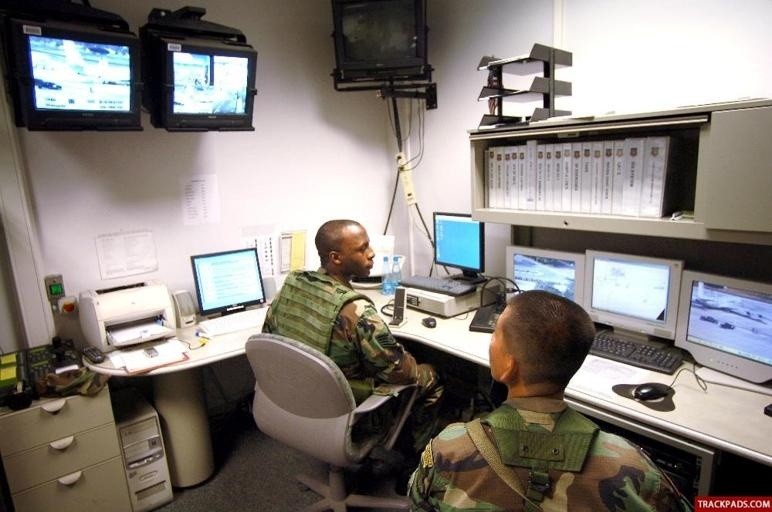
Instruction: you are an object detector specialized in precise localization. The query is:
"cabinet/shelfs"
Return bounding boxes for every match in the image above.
[470,97,772,238]
[1,385,134,512]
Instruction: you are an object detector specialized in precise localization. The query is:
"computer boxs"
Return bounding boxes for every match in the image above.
[116,390,175,512]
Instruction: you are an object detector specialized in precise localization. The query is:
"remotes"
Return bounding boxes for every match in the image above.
[82,345,104,363]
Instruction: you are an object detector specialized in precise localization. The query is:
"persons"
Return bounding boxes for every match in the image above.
[261,221,448,497]
[407,289,695,512]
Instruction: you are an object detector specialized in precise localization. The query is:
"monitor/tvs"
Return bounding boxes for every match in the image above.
[1,23,144,131]
[191,248,267,315]
[145,35,258,132]
[431,211,484,274]
[584,248,683,340]
[505,246,584,310]
[675,268,772,397]
[332,1,426,80]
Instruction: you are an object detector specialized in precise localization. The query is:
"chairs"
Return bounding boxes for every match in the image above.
[244,333,422,512]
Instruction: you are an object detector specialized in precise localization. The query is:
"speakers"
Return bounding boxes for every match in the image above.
[173,287,201,325]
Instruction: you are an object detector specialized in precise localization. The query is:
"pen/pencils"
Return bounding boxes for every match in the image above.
[194,332,212,340]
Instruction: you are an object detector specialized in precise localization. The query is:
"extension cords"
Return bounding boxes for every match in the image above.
[397,153,418,207]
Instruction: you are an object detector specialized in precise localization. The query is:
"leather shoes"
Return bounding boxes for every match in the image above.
[395,466,415,496]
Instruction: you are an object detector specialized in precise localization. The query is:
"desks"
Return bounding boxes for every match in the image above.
[82,287,770,469]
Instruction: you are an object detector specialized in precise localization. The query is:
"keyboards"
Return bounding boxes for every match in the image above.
[198,308,268,336]
[588,333,685,375]
[399,274,477,296]
[469,301,503,333]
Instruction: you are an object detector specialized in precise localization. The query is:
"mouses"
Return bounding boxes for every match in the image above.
[424,315,438,329]
[637,383,673,401]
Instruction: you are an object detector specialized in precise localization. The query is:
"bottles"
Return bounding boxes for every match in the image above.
[381,256,402,296]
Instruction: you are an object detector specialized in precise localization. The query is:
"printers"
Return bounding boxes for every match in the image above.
[80,279,188,374]
